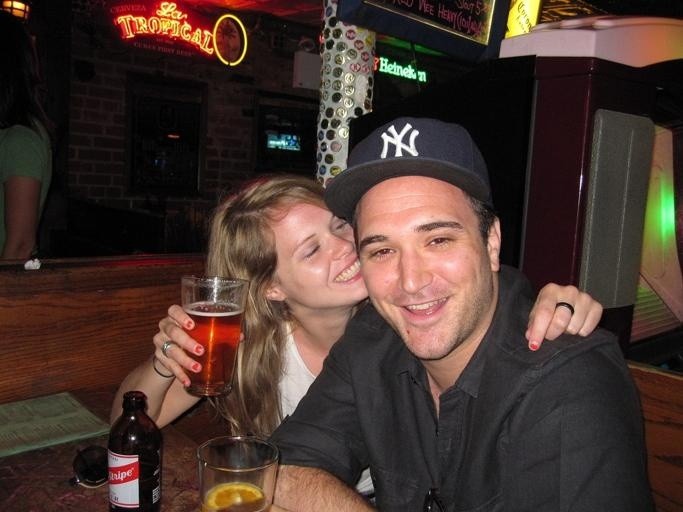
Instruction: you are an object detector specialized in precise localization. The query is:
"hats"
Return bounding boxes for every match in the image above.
[324,116,494,222]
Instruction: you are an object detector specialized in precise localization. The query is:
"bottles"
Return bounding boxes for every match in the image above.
[106,390,164,509]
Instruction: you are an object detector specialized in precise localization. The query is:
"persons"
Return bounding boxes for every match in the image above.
[0,35,53,259]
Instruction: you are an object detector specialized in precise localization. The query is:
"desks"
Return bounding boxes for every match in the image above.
[0,381,290,510]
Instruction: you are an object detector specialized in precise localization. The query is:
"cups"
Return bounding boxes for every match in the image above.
[179,276,251,399]
[196,433,280,512]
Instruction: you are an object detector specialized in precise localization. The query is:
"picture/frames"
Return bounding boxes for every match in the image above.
[122,73,208,200]
